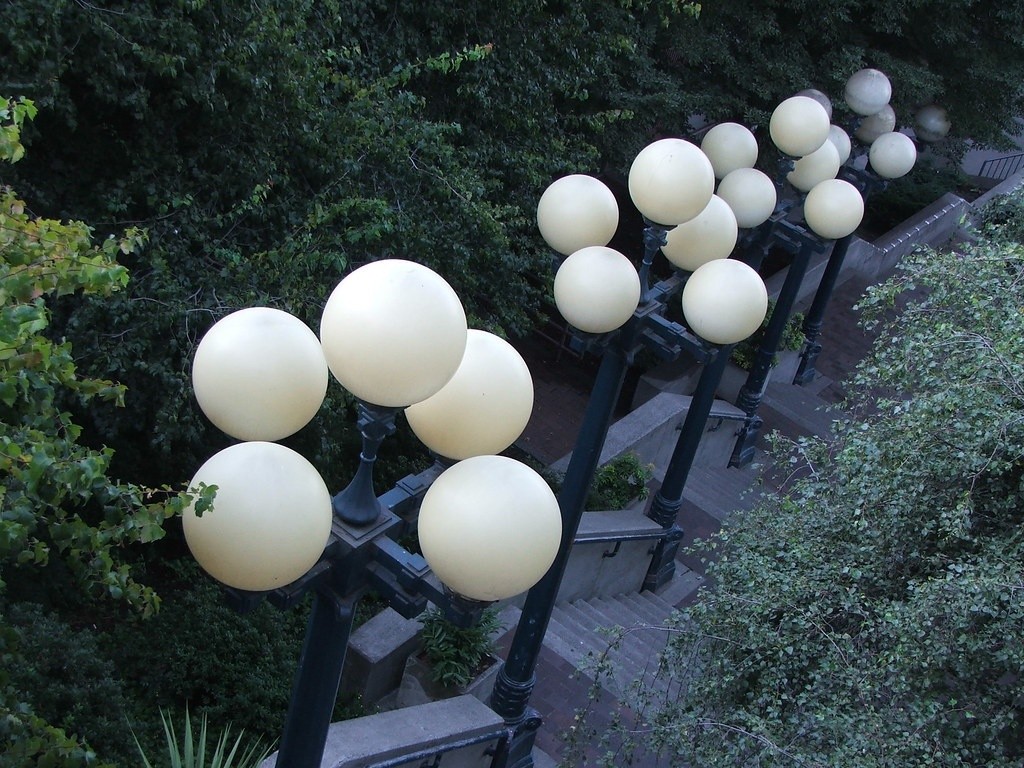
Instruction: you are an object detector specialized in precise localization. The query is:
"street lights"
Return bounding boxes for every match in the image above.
[482,68,951,768]
[183,259,563,768]
